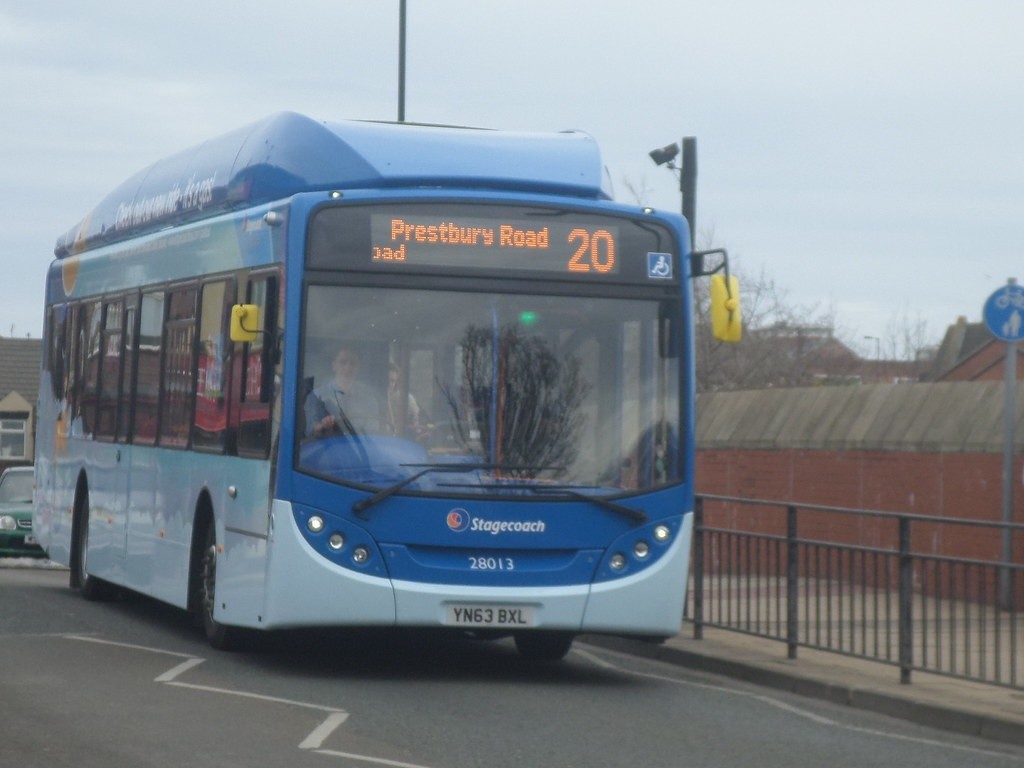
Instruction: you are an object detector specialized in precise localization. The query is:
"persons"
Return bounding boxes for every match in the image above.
[385,363,422,438]
[303,347,382,443]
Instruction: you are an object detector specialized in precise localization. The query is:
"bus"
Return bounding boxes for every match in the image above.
[31,113,740,661]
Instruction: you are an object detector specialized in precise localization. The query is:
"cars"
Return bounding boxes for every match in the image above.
[0,468,53,559]
